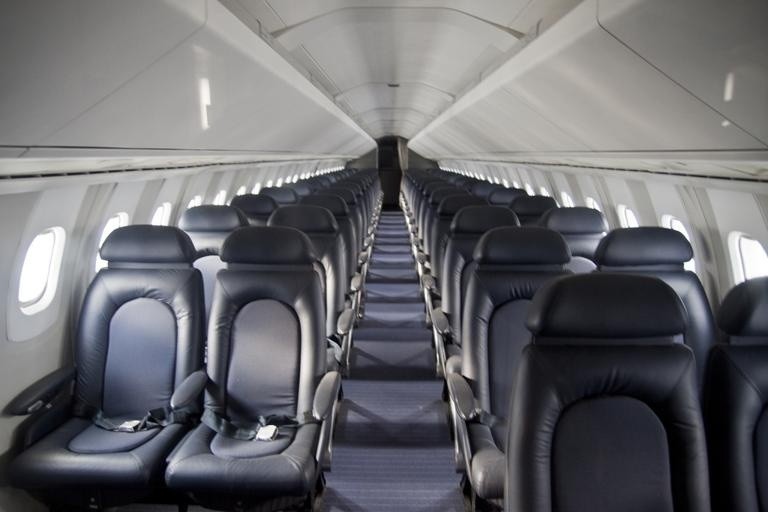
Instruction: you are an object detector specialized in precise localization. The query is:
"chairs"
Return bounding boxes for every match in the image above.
[398,165,767,512]
[0,165,385,512]
